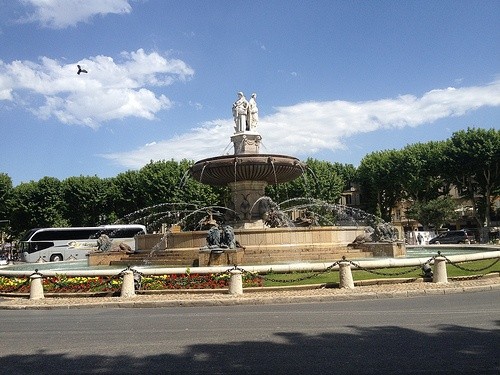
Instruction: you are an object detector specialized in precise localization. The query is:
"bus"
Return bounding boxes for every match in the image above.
[18,224,147,263]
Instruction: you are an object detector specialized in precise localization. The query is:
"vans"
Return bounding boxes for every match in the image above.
[429,231,476,244]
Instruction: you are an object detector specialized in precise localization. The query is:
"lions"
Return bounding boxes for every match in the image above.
[88,234,132,253]
[352,222,403,243]
[199,224,246,250]
